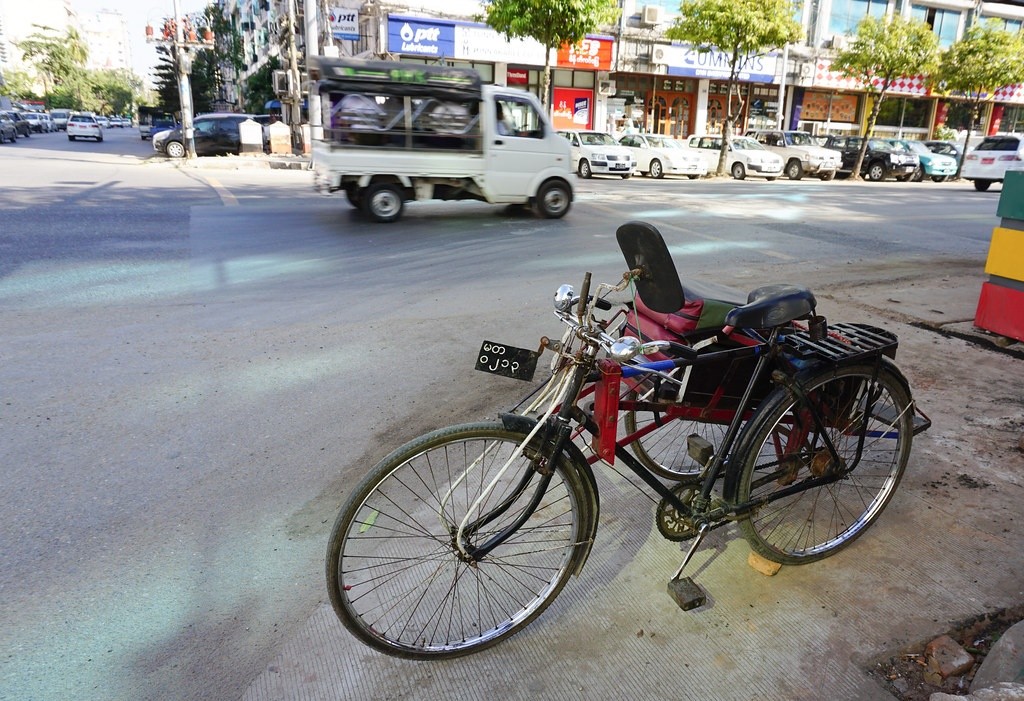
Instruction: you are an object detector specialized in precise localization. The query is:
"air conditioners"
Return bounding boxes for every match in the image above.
[639,3,665,26]
[796,62,816,79]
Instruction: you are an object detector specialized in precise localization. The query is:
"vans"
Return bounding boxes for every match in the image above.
[138,105,162,139]
[960,134,1024,191]
[685,133,784,181]
[152,112,256,152]
[813,134,920,182]
[156,117,265,156]
[52,111,71,130]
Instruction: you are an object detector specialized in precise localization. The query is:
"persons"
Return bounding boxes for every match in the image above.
[327,90,478,149]
[496,101,514,137]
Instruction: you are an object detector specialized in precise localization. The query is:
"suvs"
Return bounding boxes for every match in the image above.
[742,128,843,181]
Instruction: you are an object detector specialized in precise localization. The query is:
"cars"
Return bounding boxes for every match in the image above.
[924,141,975,180]
[0,111,18,144]
[42,114,58,131]
[122,118,133,127]
[67,114,104,141]
[96,116,110,129]
[618,133,709,179]
[22,112,49,132]
[8,111,32,137]
[554,129,638,179]
[110,117,124,127]
[886,138,958,182]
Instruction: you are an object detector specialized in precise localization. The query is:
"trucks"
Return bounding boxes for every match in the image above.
[310,52,577,221]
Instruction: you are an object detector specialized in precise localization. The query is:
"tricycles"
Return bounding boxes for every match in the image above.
[325,220,933,660]
